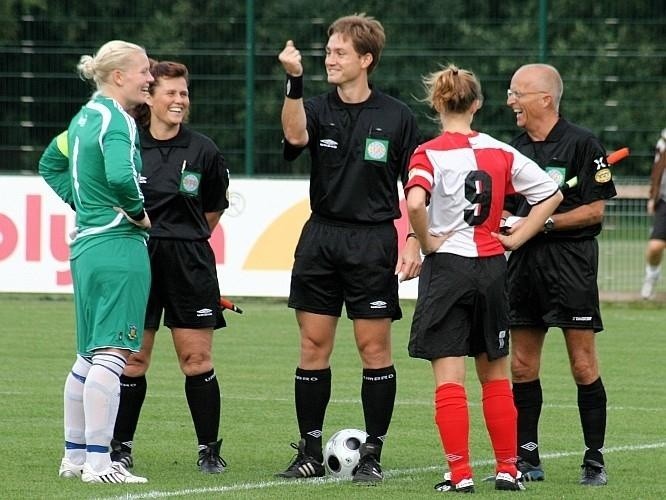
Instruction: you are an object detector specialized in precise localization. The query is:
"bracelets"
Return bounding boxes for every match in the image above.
[285,73,303,99]
[406,233,417,241]
[500,218,506,222]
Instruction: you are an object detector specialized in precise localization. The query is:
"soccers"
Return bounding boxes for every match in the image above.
[324,429,368,477]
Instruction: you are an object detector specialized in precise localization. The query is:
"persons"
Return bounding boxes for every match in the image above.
[404,64,565,493]
[481,63,617,485]
[275,13,429,483]
[110,61,230,475]
[640,128,666,300]
[38,40,155,484]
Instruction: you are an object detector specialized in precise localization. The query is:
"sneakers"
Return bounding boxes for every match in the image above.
[354,443,384,482]
[581,460,608,485]
[434,456,544,493]
[274,439,325,478]
[59,440,148,484]
[197,438,226,473]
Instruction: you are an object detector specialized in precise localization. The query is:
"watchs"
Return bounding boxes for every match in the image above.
[544,217,554,234]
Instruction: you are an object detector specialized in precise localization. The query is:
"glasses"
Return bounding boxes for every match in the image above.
[507,89,548,97]
